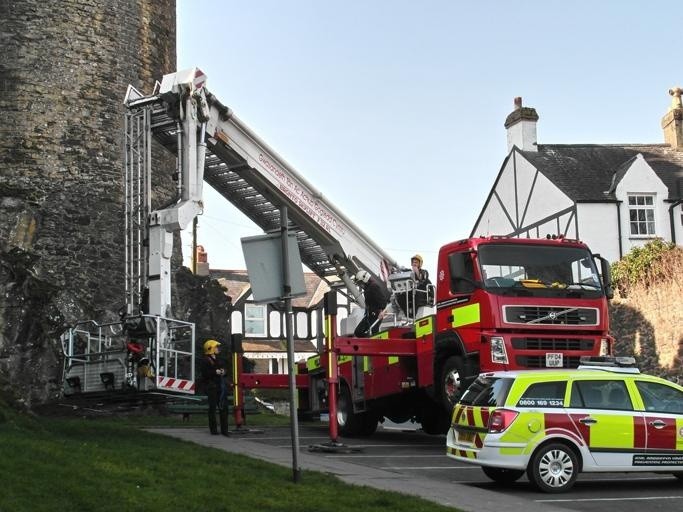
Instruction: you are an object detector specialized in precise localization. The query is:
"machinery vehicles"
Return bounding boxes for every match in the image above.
[57,67,616,438]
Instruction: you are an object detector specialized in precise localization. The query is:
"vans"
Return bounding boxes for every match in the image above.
[445,367,683,494]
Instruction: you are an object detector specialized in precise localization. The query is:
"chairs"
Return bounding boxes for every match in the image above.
[584,388,629,408]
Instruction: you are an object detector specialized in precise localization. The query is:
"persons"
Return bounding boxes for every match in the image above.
[397,255,433,318]
[202,339,231,437]
[354,271,387,338]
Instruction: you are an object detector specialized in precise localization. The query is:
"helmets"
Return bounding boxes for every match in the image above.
[203,340,222,355]
[411,255,423,266]
[355,271,371,283]
